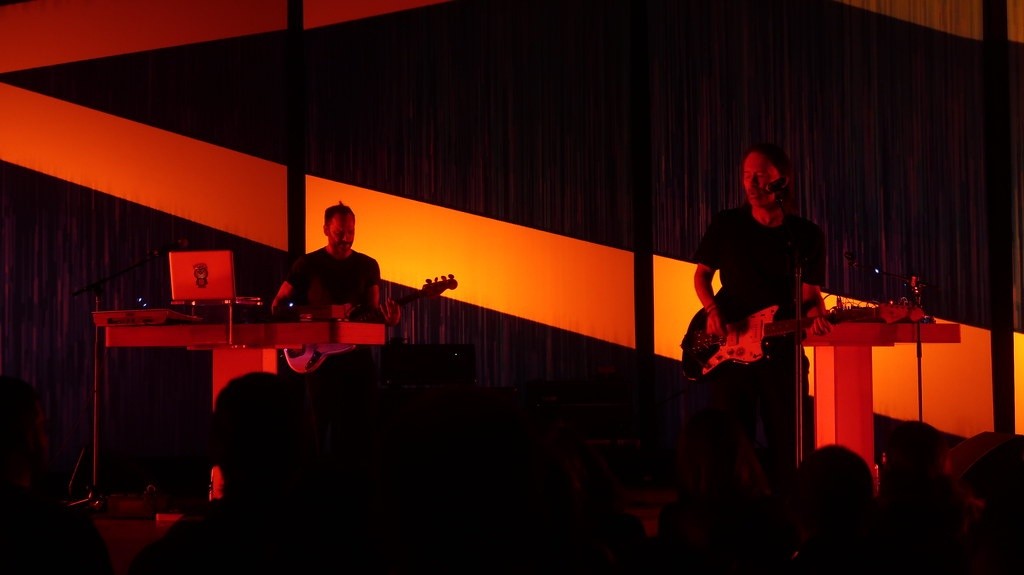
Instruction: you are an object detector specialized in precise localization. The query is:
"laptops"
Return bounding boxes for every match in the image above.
[169,251,261,301]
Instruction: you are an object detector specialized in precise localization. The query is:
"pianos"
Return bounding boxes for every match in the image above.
[104,322,386,348]
[798,323,962,347]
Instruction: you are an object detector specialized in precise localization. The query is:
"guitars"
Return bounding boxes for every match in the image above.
[681,296,913,382]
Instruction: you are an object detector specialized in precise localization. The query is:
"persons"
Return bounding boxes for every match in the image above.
[127,375,1024,575]
[694,144,831,491]
[272,204,402,450]
[0,375,113,575]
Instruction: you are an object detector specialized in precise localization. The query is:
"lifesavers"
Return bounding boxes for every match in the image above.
[282,273,457,373]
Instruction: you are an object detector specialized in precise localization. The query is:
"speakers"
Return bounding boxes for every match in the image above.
[368,385,525,501]
[942,430,1024,517]
[536,405,640,506]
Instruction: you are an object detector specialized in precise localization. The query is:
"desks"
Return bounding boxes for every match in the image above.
[105,322,387,503]
[802,322,961,497]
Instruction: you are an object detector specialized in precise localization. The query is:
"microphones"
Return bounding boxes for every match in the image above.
[766,176,789,192]
[154,239,188,256]
